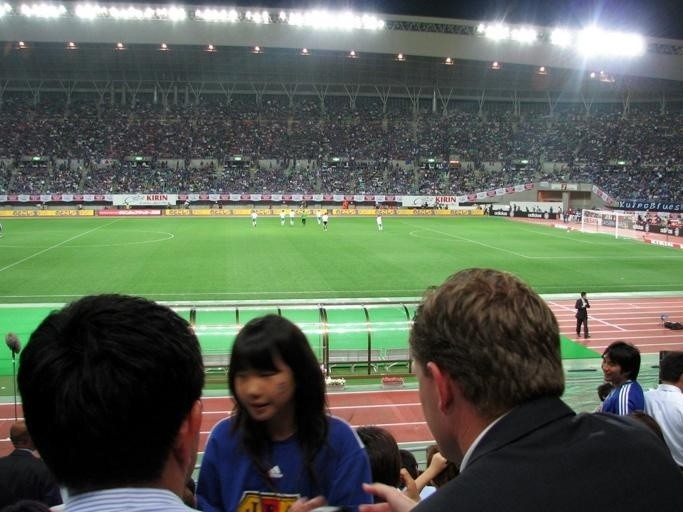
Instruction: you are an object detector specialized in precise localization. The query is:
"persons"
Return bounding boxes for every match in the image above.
[573,291,593,339]
[0,96,682,227]
[642,352,682,469]
[594,340,644,418]
[320,212,329,232]
[0,420,68,512]
[594,382,614,412]
[196,313,374,512]
[352,263,683,512]
[375,214,383,231]
[356,425,460,503]
[15,290,208,512]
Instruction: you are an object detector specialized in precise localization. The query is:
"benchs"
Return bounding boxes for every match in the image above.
[200,348,414,375]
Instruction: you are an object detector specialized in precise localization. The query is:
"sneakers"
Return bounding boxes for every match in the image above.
[584,335,591,339]
[577,332,581,337]
